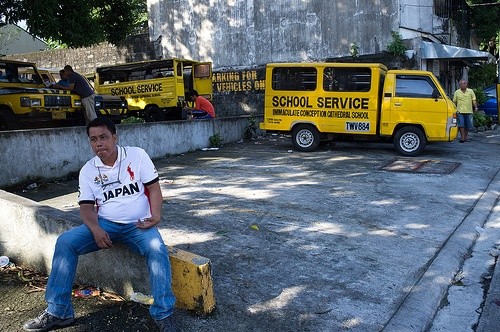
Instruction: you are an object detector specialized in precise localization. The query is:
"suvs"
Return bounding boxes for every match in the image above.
[1,59,82,128]
[38,69,128,124]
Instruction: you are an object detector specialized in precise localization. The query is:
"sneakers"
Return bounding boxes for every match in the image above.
[154,314,184,332]
[23,311,75,332]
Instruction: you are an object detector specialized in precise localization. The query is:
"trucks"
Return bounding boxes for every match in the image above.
[86,57,214,124]
[259,63,458,156]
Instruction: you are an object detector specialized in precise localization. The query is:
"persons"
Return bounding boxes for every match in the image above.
[22,116,179,332]
[453,79,478,143]
[182,90,215,120]
[50,66,97,121]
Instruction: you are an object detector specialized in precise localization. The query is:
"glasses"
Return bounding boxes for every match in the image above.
[101,180,122,190]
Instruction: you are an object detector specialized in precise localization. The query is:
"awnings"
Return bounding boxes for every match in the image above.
[419,41,497,62]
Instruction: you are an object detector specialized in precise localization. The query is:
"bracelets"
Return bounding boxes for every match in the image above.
[475,108,478,109]
